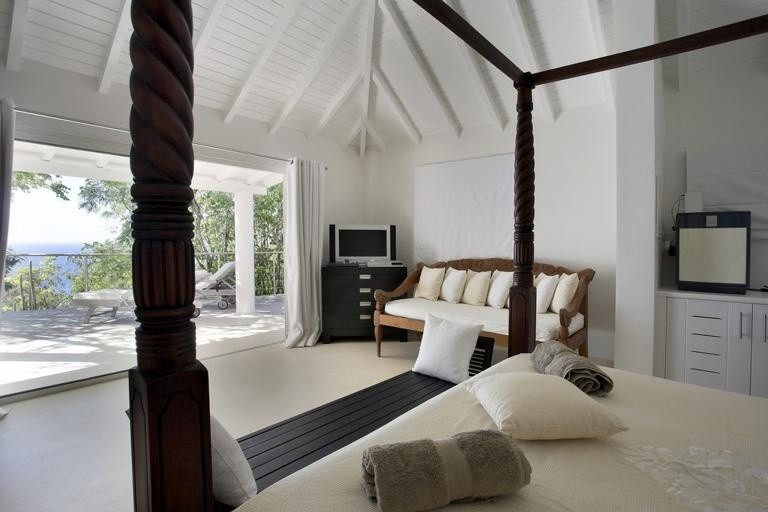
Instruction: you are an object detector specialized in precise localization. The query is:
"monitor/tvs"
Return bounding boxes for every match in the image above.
[328,224,396,268]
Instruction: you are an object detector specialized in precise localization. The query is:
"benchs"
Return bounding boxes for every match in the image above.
[214,335,495,512]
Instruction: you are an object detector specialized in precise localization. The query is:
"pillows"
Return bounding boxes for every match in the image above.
[486,269,514,308]
[439,267,466,304]
[411,312,484,385]
[463,373,630,439]
[548,272,579,314]
[414,265,445,301]
[505,274,536,308]
[209,414,258,507]
[460,269,491,306]
[534,272,561,313]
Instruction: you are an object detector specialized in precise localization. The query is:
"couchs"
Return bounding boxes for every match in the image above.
[371,258,596,358]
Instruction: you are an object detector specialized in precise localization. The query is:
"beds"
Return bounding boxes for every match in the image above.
[129,0,768,512]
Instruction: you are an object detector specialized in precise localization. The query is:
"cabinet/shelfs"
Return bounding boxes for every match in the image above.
[321,263,407,342]
[666,296,768,399]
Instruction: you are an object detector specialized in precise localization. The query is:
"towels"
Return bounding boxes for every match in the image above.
[359,427,532,512]
[530,340,613,398]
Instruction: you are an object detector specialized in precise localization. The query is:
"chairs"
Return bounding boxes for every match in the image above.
[73,260,235,323]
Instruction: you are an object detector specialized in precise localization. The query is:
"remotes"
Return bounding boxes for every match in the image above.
[326,261,359,268]
